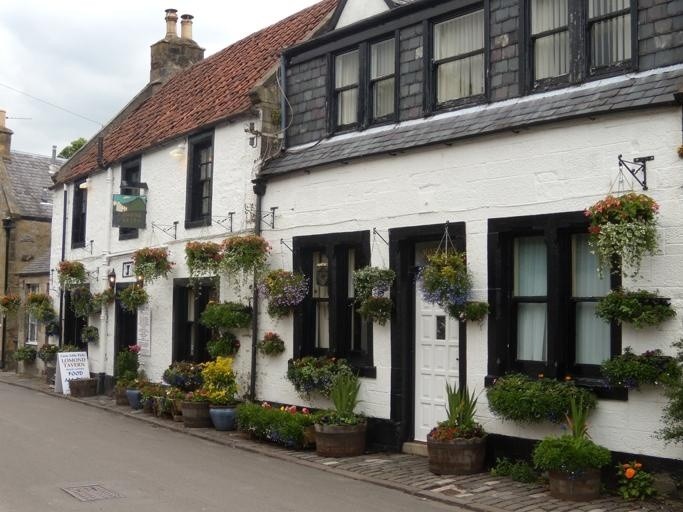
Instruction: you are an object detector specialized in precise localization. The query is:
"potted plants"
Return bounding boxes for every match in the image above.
[534,438,611,501]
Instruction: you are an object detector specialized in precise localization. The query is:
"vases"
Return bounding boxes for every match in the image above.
[126,387,238,432]
[66,376,97,397]
[315,422,366,456]
[424,433,487,473]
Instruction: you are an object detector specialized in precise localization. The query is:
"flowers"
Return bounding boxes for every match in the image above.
[314,371,367,426]
[414,249,470,308]
[115,235,310,404]
[583,193,662,279]
[424,383,487,440]
[351,265,395,306]
